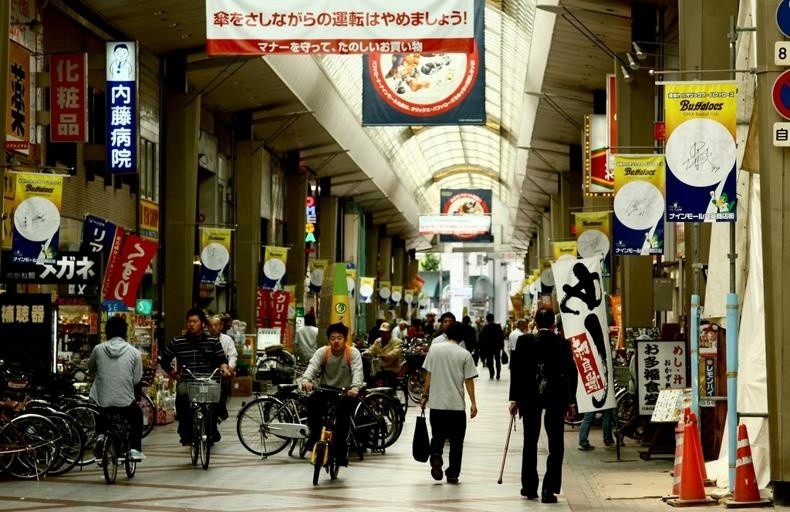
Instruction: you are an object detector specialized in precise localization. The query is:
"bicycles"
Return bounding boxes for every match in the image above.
[562,325,656,442]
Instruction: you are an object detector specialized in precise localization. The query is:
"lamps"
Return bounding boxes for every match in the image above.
[618,36,680,84]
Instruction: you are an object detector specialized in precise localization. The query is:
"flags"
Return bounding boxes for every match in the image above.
[548,256,619,415]
[105,232,161,308]
[611,151,666,257]
[308,258,428,310]
[197,226,233,284]
[656,79,740,223]
[518,237,576,300]
[572,210,614,279]
[261,245,288,291]
[11,171,63,261]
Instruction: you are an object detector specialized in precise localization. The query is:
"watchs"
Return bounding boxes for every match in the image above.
[420,393,431,399]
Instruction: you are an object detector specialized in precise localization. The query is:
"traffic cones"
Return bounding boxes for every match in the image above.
[663,409,717,508]
[725,423,775,509]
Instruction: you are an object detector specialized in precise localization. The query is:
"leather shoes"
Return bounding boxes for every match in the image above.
[541,488,560,503]
[520,486,539,499]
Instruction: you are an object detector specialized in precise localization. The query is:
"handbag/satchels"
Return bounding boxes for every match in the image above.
[411,408,432,464]
[501,351,509,365]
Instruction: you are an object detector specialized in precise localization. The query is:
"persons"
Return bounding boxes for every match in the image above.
[430,312,467,443]
[358,321,405,415]
[208,311,238,423]
[366,312,529,380]
[625,351,648,436]
[296,320,365,466]
[505,307,577,502]
[579,409,623,454]
[84,315,146,462]
[293,313,319,364]
[419,319,477,486]
[157,308,231,446]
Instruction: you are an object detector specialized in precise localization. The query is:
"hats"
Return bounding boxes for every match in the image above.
[379,322,393,333]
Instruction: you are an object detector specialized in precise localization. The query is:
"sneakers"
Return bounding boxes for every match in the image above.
[92,434,106,458]
[605,441,620,450]
[335,453,348,467]
[430,453,443,481]
[578,439,595,451]
[445,469,460,484]
[306,429,321,452]
[177,425,224,446]
[124,448,147,460]
[475,363,502,380]
